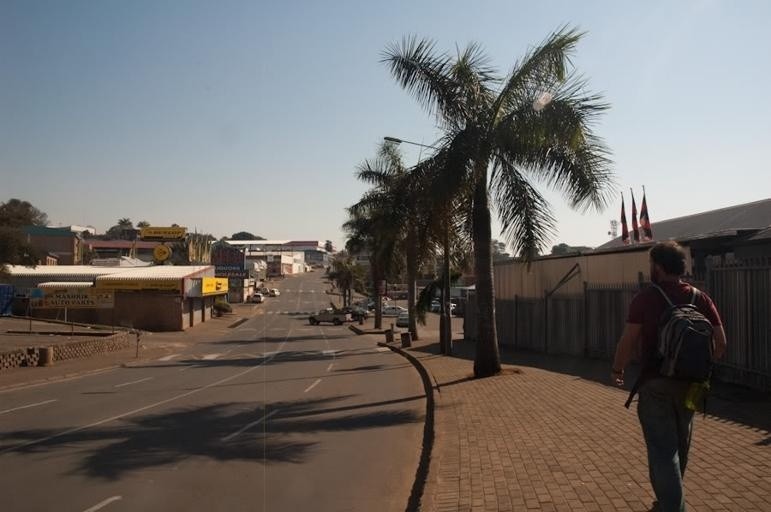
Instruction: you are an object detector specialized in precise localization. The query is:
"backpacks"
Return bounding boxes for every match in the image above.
[650,283,715,393]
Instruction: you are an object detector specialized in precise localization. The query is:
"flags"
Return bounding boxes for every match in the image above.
[620,193,632,247]
[640,190,654,242]
[631,192,642,245]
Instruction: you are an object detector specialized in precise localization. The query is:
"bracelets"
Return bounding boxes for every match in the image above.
[612,367,626,376]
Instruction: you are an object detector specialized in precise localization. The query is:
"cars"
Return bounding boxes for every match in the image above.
[252,287,280,304]
[339,294,409,327]
[427,299,456,313]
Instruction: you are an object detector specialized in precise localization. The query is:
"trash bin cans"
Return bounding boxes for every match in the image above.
[39,347,53,366]
[400,332,412,347]
[385,330,393,343]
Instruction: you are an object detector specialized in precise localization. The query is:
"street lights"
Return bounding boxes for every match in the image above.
[382,135,455,357]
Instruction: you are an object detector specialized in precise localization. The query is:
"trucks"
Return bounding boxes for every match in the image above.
[309,308,352,325]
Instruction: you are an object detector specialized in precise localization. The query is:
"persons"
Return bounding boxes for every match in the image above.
[610,241,730,511]
